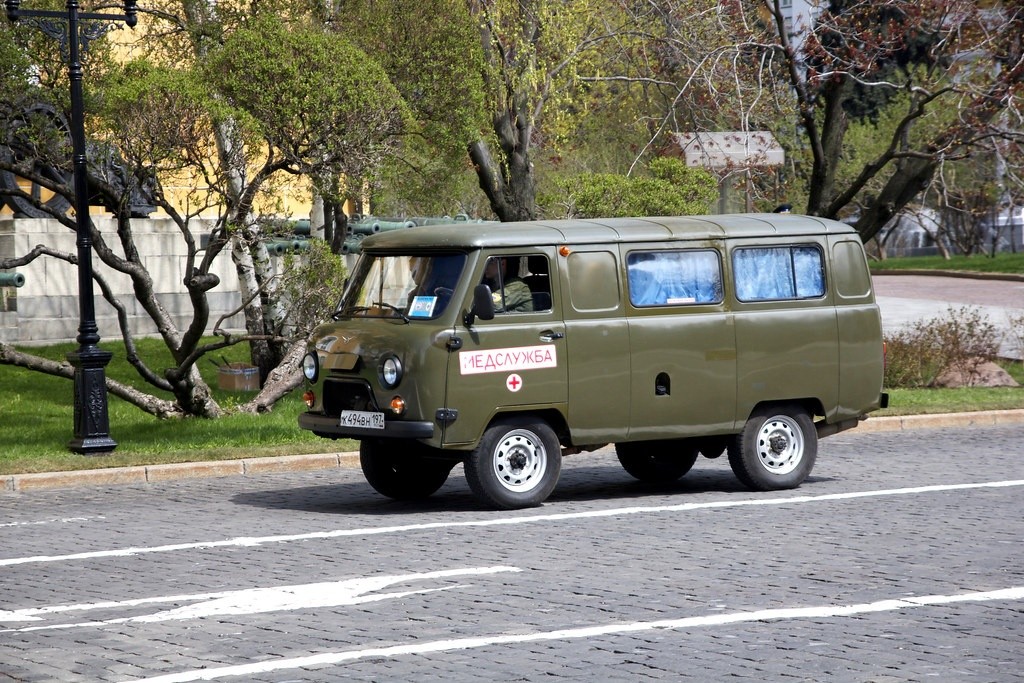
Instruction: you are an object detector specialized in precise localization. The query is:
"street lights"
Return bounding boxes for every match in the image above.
[6,0,137,454]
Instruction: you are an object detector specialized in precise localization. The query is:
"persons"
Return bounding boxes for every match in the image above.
[401,256,450,317]
[472,257,533,312]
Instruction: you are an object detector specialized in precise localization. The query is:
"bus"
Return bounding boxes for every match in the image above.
[297,202,889,514]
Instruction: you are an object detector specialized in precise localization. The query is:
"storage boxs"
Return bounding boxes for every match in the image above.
[216,362,260,392]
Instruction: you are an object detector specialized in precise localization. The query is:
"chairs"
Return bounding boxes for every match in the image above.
[531,291,551,311]
[524,256,549,293]
